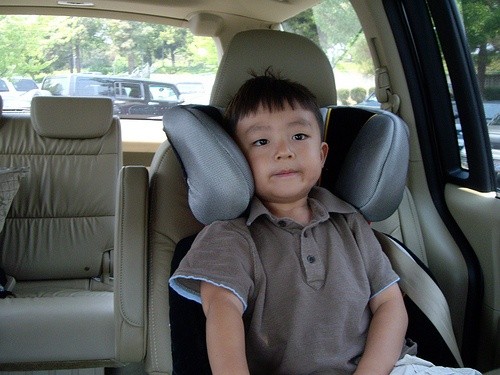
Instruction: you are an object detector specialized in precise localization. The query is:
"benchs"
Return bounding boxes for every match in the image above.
[0,96,127,364]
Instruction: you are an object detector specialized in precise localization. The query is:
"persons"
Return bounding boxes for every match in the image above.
[168,65,484,375]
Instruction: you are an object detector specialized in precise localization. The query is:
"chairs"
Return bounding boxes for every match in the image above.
[113,29,429,375]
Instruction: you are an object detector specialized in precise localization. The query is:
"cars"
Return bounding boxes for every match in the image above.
[0,76,43,110]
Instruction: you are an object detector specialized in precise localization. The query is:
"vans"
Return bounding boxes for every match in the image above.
[41,73,186,117]
